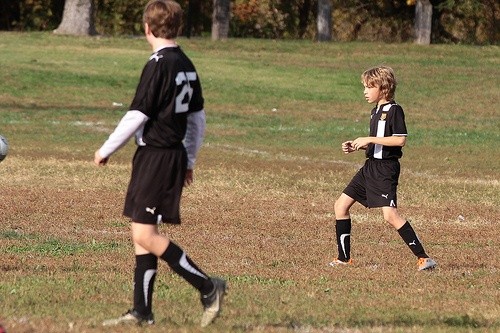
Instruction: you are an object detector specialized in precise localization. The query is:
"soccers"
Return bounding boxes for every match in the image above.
[0,135,8,164]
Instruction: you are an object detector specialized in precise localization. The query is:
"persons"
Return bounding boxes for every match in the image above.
[93,0,228,330]
[324,64,438,273]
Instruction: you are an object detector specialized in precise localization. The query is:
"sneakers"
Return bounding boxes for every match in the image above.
[103,308,155,327]
[417,257,436,271]
[200,278,227,328]
[325,258,353,267]
